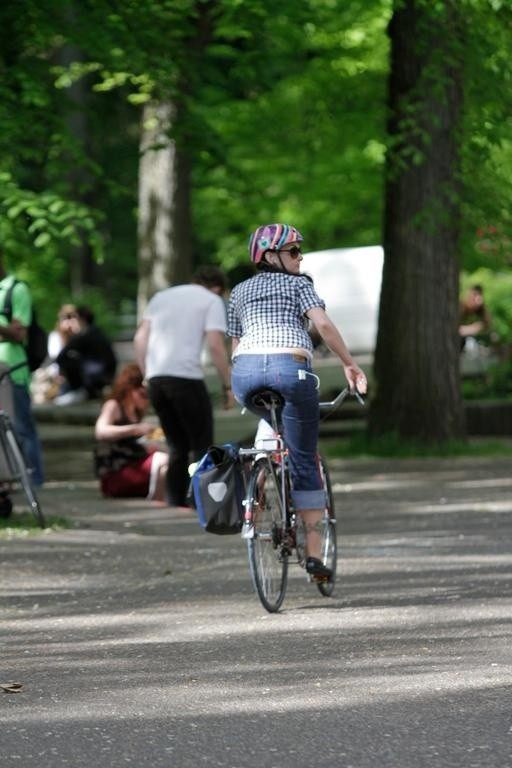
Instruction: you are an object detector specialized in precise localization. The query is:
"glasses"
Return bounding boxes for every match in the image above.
[270,247,303,260]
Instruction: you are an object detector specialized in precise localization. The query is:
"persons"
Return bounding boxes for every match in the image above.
[0,265,44,489]
[132,263,234,509]
[459,282,494,355]
[222,222,369,582]
[50,302,118,407]
[94,362,171,500]
[45,303,84,360]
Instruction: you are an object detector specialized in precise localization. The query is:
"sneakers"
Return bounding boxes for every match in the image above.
[305,556,335,580]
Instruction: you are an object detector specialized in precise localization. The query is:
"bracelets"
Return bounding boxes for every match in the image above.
[223,383,233,391]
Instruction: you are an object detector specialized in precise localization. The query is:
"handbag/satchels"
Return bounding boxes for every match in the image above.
[186,441,248,536]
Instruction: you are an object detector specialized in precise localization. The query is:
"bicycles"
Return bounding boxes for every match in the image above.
[238,385,366,613]
[0,357,46,528]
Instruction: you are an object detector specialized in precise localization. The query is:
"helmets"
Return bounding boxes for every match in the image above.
[248,223,304,265]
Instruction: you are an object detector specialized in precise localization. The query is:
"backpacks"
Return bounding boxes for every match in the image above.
[3,276,48,372]
